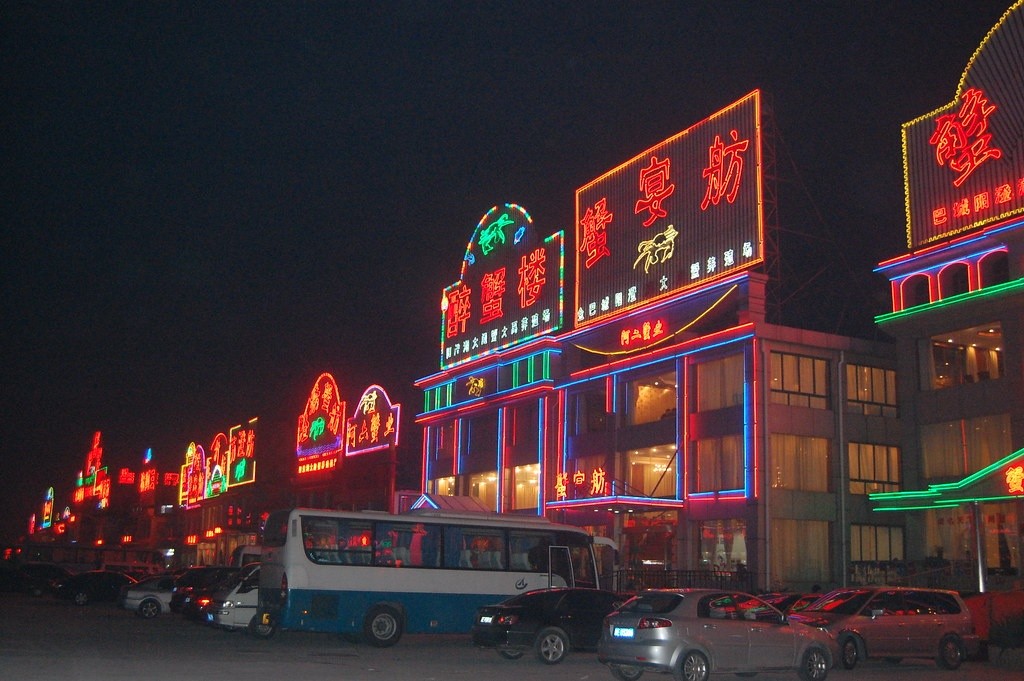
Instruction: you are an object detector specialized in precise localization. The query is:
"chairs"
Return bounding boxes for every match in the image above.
[481,551,492,568]
[511,553,530,570]
[708,607,727,618]
[461,550,473,568]
[492,551,505,569]
[343,552,352,564]
[331,545,342,563]
[353,546,372,565]
[390,547,411,566]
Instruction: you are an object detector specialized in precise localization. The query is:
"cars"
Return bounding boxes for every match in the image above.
[18,562,89,608]
[737,592,827,626]
[474,587,634,665]
[599,590,837,681]
[74,570,141,607]
[124,575,182,618]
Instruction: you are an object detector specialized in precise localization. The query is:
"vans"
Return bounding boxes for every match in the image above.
[232,544,265,569]
[101,562,162,582]
[209,560,267,632]
[789,586,981,669]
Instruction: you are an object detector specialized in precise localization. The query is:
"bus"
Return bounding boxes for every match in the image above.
[258,505,621,647]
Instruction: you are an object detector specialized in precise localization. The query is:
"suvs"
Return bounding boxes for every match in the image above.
[171,564,242,622]
